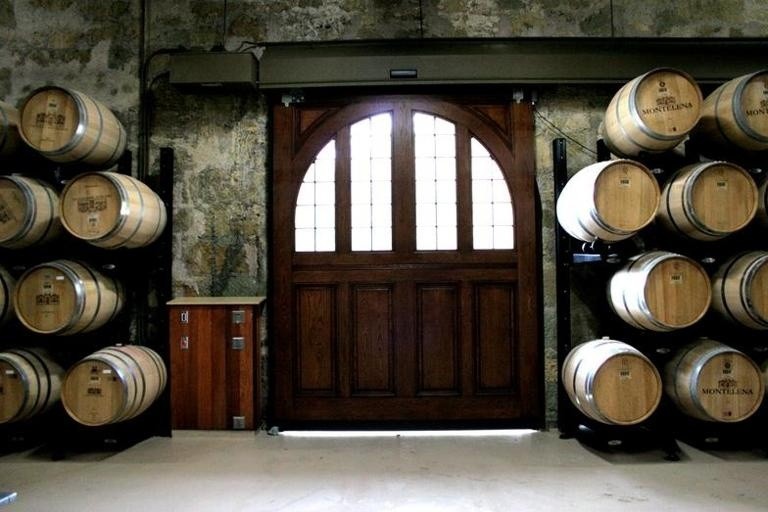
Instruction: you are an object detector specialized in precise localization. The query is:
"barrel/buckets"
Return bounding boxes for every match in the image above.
[600,67,704,158]
[12,259,127,337]
[605,249,713,332]
[0,268,18,327]
[15,83,127,170]
[555,158,662,246]
[710,249,768,332]
[59,343,170,427]
[57,171,168,251]
[756,174,768,229]
[0,175,64,251]
[695,69,768,151]
[650,160,758,243]
[0,100,22,155]
[560,336,664,427]
[0,347,67,425]
[760,357,768,396]
[658,337,765,423]
